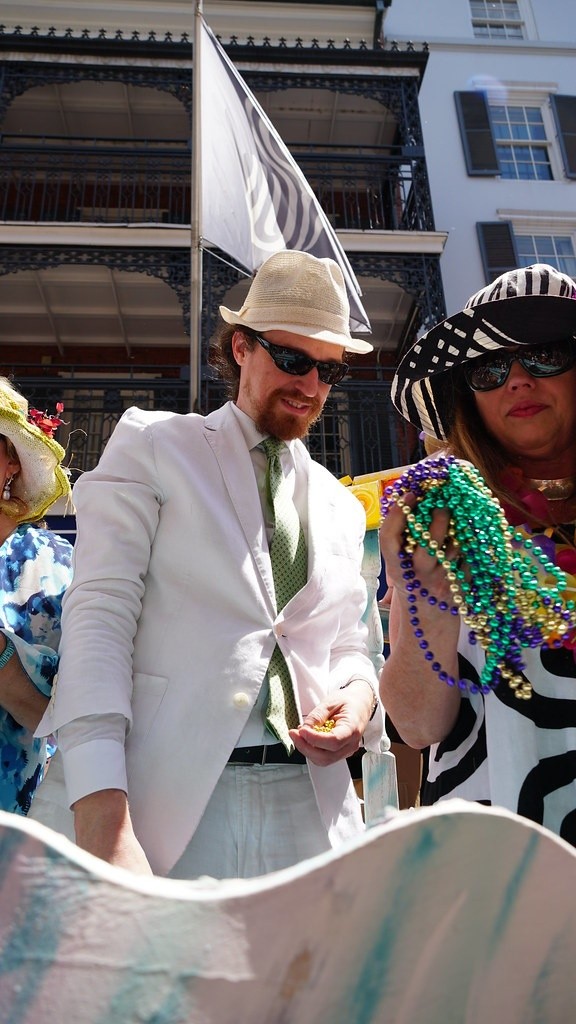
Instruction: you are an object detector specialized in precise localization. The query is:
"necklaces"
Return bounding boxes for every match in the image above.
[378,456,576,700]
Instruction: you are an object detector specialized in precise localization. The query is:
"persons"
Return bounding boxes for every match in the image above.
[0,375,75,816]
[24,247,391,882]
[378,260,576,843]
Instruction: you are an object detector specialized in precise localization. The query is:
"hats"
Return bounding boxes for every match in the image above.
[390,263,576,444]
[0,381,71,523]
[220,249,375,355]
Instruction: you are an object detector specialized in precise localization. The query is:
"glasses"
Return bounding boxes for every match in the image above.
[253,332,349,385]
[460,336,576,392]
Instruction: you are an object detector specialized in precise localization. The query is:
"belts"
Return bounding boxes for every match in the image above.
[228,743,306,764]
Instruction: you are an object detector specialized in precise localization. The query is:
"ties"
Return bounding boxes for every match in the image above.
[263,436,309,758]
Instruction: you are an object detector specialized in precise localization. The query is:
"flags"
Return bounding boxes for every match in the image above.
[199,12,375,336]
